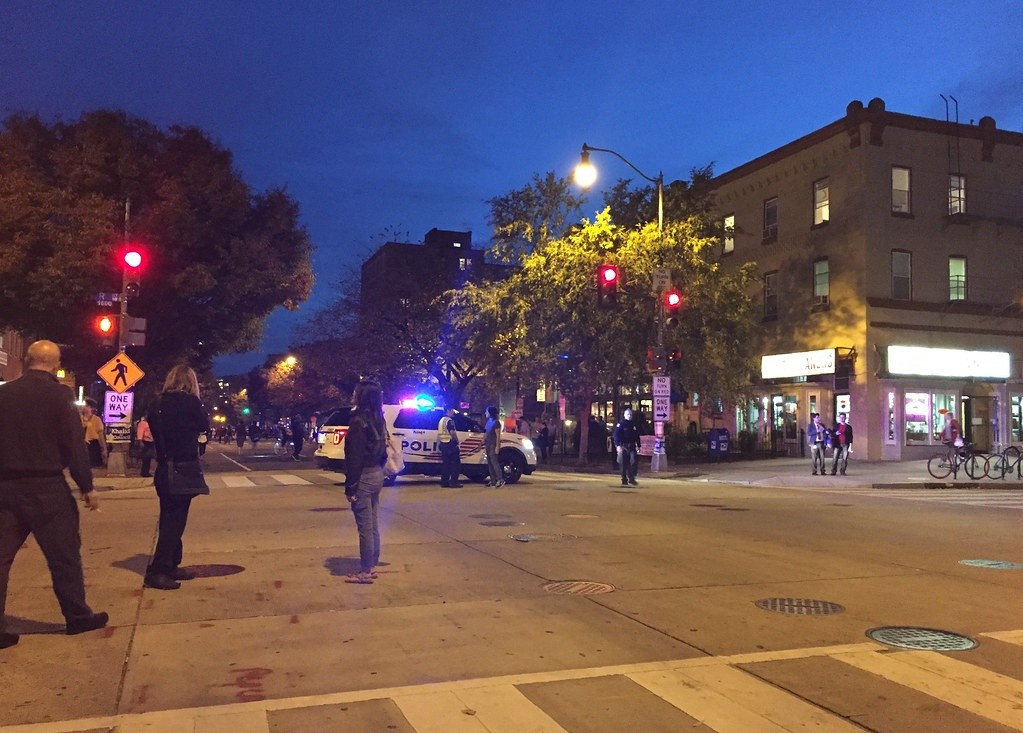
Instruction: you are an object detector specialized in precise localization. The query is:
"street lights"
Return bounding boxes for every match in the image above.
[573,144,669,473]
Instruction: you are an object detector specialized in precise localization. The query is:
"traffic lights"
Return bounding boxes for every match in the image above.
[123,249,143,296]
[121,315,147,347]
[662,291,680,330]
[96,314,119,349]
[667,347,681,368]
[647,344,664,370]
[598,265,616,309]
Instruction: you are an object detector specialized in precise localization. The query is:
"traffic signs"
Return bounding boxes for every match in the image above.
[104,391,134,423]
[653,395,670,421]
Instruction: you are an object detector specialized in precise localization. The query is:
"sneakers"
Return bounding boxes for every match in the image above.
[485,481,496,487]
[496,479,506,488]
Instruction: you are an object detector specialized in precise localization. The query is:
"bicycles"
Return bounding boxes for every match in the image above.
[273,437,295,457]
[984,444,1023,479]
[928,440,991,480]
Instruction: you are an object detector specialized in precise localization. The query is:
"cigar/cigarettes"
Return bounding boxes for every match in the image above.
[96,508,101,512]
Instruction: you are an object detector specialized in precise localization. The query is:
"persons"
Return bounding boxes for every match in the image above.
[344,380,389,585]
[207,414,317,461]
[807,413,827,475]
[80,407,155,477]
[501,416,607,464]
[938,412,961,467]
[829,412,853,475]
[614,408,641,487]
[0,340,109,649]
[437,405,462,487]
[145,364,210,591]
[483,407,505,488]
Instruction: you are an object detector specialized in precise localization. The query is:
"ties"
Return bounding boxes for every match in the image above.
[817,425,822,440]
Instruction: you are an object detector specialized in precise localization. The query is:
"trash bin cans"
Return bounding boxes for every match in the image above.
[708,427,729,458]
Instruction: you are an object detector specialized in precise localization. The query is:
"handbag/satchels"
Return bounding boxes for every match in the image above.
[383,438,405,475]
[167,439,199,474]
[954,433,965,446]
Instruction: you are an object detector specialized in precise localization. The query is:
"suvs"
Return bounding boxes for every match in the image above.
[314,398,537,484]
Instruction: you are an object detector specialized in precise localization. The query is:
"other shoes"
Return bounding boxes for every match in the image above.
[140,473,153,477]
[65,612,109,635]
[821,473,827,475]
[0,633,19,649]
[812,473,818,475]
[441,482,463,488]
[170,568,196,580]
[291,455,301,462]
[345,570,378,584]
[144,574,181,589]
[622,482,628,485]
[629,480,637,485]
[831,472,836,475]
[841,473,847,476]
